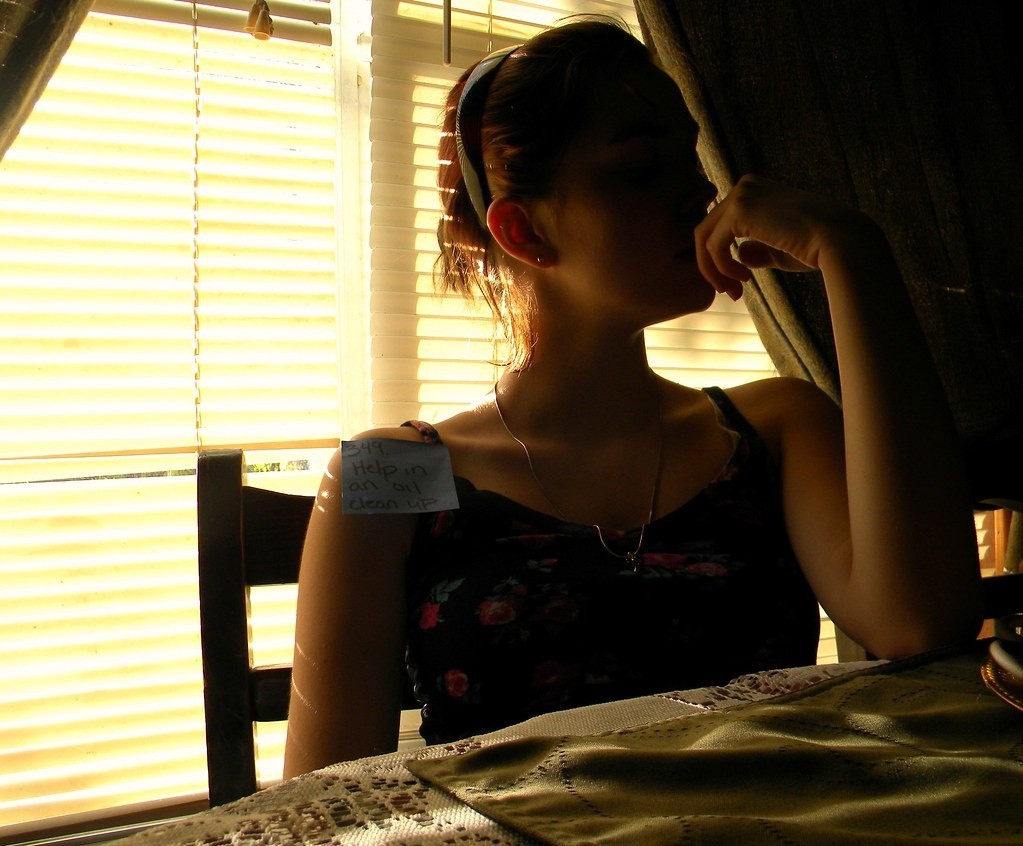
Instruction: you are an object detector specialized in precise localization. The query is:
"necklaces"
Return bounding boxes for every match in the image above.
[494,369,665,574]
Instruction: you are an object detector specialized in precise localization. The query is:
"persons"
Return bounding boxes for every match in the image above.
[285,18,987,779]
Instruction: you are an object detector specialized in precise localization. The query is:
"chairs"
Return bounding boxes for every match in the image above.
[195,455,424,809]
